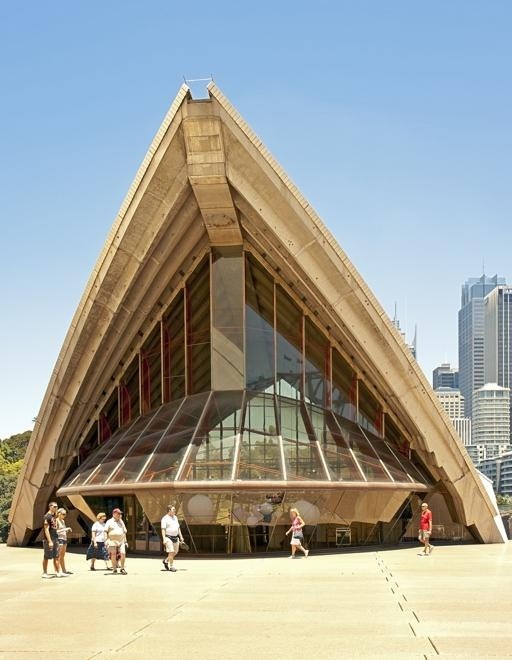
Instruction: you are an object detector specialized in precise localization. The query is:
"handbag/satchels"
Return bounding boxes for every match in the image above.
[294,531,303,539]
[167,535,179,542]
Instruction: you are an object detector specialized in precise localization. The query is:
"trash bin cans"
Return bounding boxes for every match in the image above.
[336,528,351,545]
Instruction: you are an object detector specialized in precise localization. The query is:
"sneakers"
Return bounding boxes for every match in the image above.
[305,550,309,558]
[419,545,434,556]
[288,555,295,559]
[42,570,73,578]
[163,560,177,572]
[120,569,127,575]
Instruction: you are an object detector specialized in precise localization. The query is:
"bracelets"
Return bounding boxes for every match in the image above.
[429,529,432,531]
[124,542,127,544]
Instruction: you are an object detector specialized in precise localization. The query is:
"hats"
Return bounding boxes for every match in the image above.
[113,508,123,513]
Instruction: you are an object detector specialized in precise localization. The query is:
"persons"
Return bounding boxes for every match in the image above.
[417,503,434,556]
[104,508,129,575]
[87,512,113,570]
[161,505,184,571]
[55,508,75,574]
[36,501,67,578]
[105,512,123,568]
[286,507,309,559]
